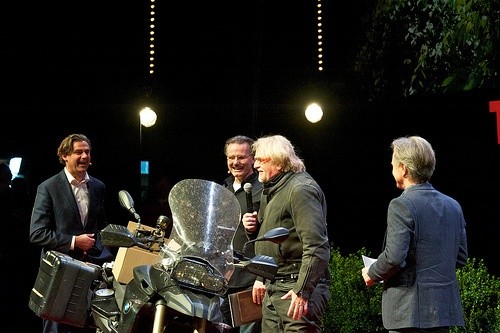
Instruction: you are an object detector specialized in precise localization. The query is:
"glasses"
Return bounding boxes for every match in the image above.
[254,158,271,164]
[226,153,252,162]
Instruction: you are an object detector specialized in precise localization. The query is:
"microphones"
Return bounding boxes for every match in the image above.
[243,182,254,214]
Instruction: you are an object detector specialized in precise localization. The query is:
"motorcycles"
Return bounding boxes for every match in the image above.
[29,178,290,333]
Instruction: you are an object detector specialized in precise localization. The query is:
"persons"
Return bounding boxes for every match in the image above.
[0,134,115,333]
[242,135,332,333]
[221,136,265,333]
[362,137,468,333]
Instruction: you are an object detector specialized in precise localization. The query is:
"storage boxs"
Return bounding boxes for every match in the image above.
[29,251,96,328]
[111,221,170,285]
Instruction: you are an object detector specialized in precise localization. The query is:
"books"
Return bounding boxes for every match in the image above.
[228,290,262,328]
